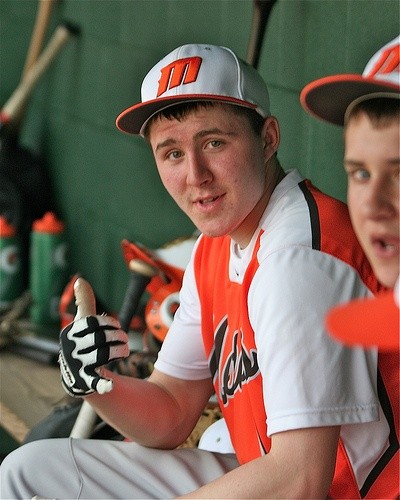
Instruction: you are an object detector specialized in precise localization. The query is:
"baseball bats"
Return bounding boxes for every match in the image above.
[67,256,159,440]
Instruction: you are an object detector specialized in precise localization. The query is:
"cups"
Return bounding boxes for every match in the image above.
[0,214,26,319]
[28,210,69,325]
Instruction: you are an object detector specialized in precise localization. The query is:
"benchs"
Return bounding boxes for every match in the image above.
[1,351,227,451]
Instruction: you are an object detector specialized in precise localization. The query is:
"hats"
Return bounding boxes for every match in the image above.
[115,43,271,138]
[299,34,399,123]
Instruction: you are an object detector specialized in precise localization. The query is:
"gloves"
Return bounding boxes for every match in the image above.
[57,277,133,398]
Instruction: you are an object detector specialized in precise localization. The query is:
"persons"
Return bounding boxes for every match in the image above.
[0,42,393,500]
[302,35,400,290]
